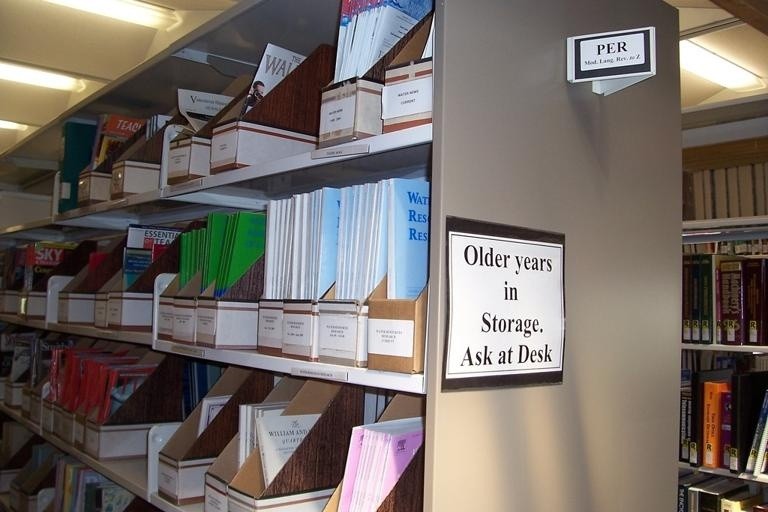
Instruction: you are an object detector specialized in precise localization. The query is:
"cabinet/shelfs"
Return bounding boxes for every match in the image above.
[680,94,767,512]
[0,0,680,512]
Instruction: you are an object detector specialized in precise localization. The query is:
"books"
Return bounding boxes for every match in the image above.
[682,239,768,345]
[58,1,435,215]
[1,178,430,300]
[693,162,768,219]
[1,322,426,512]
[680,350,768,512]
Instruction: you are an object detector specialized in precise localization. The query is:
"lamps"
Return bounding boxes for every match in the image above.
[0,120,27,133]
[677,37,764,95]
[50,0,182,30]
[0,59,86,93]
